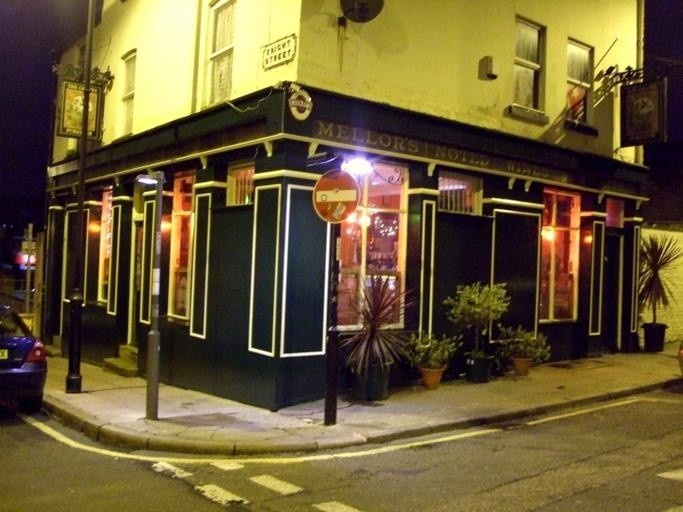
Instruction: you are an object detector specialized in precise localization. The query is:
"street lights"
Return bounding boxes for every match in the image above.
[136,171,167,419]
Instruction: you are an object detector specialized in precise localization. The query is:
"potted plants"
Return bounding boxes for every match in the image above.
[441,281,512,383]
[407,329,463,389]
[337,270,411,402]
[637,233,683,352]
[497,323,552,376]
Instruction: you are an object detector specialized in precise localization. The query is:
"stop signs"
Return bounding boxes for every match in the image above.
[312,168,360,225]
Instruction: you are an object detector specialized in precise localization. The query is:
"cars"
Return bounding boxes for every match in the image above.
[0,306,47,416]
